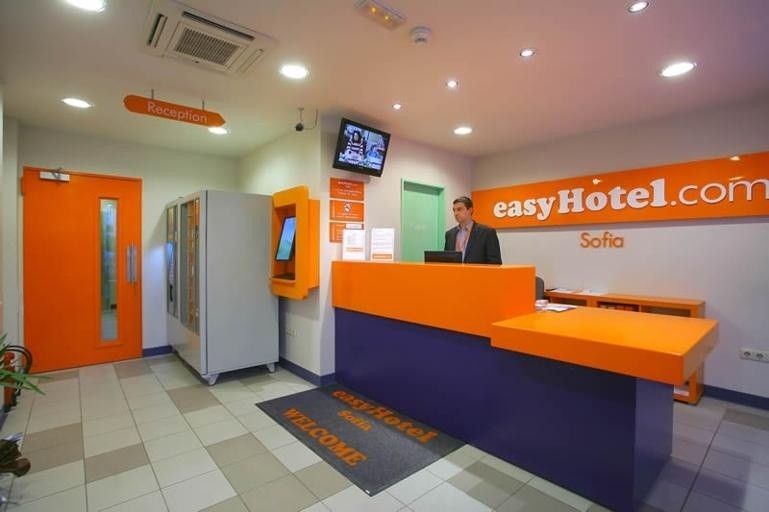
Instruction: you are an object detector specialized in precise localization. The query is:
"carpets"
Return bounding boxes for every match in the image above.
[254,381,468,498]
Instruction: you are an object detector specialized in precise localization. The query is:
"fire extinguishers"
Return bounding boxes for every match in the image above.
[0,344,32,406]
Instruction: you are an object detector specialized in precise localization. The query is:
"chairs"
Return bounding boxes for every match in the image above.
[534,275,544,301]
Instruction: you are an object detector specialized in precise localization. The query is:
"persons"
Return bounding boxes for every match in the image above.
[345,129,364,156]
[442,195,503,264]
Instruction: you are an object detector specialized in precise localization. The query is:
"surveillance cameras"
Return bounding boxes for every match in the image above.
[294,121,305,132]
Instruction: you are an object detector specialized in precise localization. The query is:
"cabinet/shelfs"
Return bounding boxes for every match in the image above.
[545,291,706,405]
[162,188,280,389]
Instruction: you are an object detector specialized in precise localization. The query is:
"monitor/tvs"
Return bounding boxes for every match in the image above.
[424,250,462,263]
[332,117,391,178]
[274,215,297,262]
